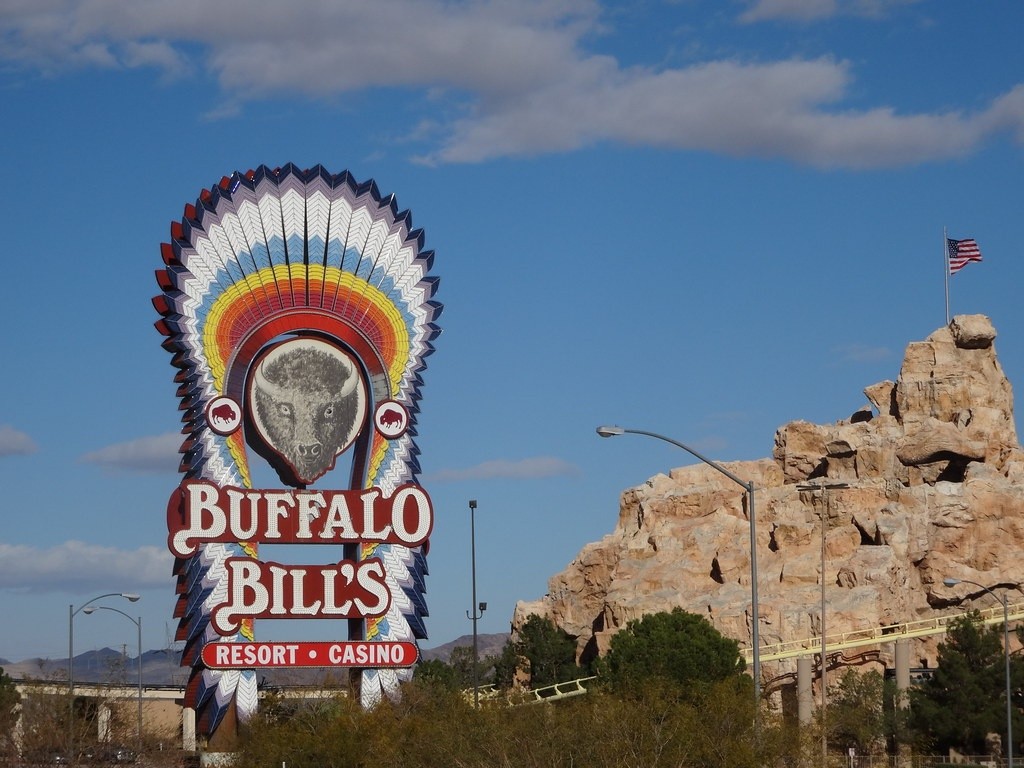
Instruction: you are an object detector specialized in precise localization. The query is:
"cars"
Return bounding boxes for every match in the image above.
[21,747,73,764]
[80,742,135,763]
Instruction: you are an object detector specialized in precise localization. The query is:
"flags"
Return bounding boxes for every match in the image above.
[947,237,983,277]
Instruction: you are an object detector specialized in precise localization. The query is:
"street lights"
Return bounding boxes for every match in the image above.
[795,478,852,768]
[68,591,141,750]
[79,604,142,746]
[464,499,490,716]
[594,424,763,750]
[941,577,1015,768]
